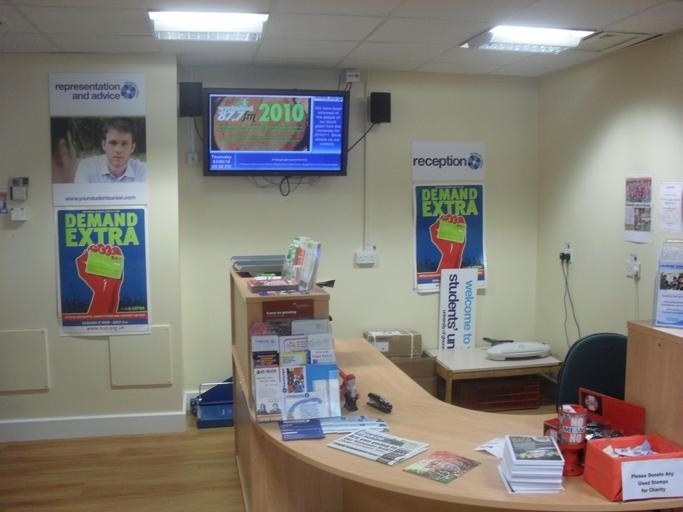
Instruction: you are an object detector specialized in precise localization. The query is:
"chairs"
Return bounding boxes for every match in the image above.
[558,332,627,410]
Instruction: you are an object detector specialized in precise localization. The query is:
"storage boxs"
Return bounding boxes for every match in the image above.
[389,356,435,377]
[362,329,423,357]
[413,375,437,398]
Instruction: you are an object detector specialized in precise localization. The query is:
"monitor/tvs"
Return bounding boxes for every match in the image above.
[202,88,350,176]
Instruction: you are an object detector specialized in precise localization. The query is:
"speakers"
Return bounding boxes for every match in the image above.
[179,82,202,117]
[370,92,391,123]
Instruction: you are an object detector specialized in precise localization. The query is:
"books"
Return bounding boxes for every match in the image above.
[247,277,299,291]
[495,433,565,494]
[279,418,326,441]
[402,448,483,485]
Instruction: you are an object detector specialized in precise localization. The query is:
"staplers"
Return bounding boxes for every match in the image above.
[366,392,392,413]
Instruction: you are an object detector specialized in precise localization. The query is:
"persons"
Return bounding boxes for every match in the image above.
[50,116,78,184]
[73,117,147,184]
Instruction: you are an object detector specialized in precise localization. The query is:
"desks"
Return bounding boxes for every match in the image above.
[420,346,563,405]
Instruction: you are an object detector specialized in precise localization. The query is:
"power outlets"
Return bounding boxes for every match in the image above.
[626,261,641,277]
[354,250,378,264]
[562,248,573,263]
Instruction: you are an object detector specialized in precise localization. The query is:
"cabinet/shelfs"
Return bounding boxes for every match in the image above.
[624,317,682,447]
